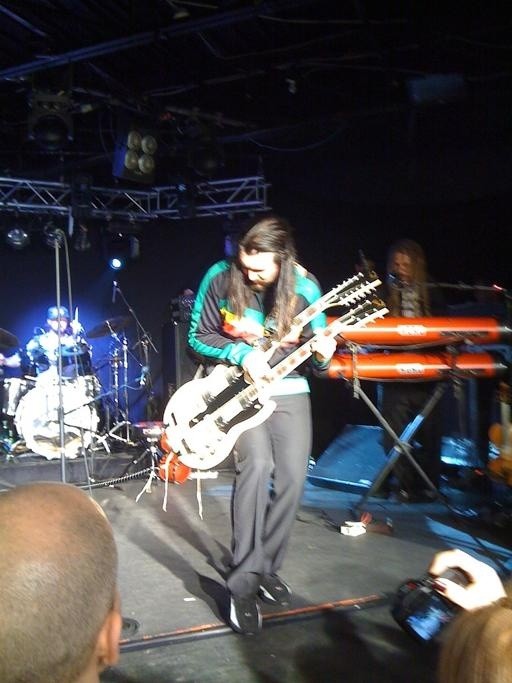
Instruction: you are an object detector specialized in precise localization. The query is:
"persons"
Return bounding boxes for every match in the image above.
[0,305,92,382]
[380,237,450,503]
[189,216,337,636]
[427,547,511,681]
[1,481,121,682]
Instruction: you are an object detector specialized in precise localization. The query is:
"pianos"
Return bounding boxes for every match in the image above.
[325,316,500,383]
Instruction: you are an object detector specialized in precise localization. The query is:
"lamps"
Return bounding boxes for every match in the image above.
[3,73,225,248]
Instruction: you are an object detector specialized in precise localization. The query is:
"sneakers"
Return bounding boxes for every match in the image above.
[258,575,291,606]
[229,596,263,636]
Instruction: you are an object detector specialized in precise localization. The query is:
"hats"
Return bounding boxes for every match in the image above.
[48,306,69,319]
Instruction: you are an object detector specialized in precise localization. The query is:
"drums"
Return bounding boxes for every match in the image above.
[14,385,98,459]
[0,377,36,416]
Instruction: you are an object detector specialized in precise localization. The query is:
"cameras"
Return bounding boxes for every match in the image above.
[390,567,471,646]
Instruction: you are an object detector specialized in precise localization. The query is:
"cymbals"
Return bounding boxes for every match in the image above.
[1,329,19,346]
[86,317,131,338]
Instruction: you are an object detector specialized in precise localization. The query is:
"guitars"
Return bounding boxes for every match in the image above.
[489,382,511,482]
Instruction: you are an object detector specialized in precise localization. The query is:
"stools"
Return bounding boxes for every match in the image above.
[131,420,169,495]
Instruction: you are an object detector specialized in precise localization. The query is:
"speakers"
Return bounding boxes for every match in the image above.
[305,423,419,495]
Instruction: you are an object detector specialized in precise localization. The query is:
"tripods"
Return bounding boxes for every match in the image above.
[135,339,174,503]
[84,346,148,457]
